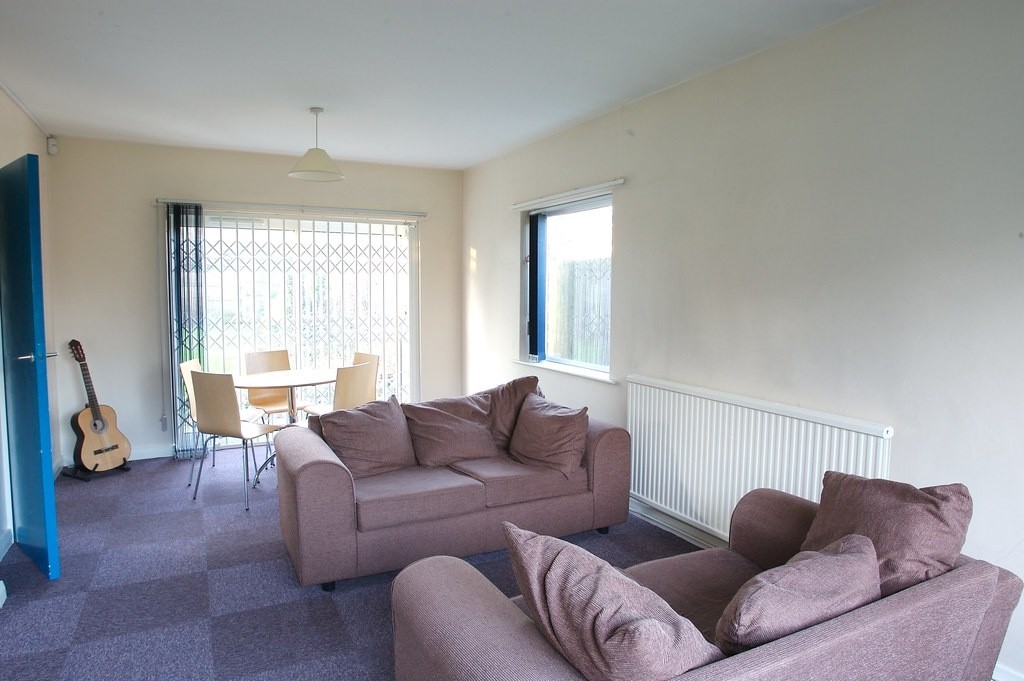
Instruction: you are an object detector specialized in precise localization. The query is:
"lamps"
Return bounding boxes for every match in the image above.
[289,106,347,184]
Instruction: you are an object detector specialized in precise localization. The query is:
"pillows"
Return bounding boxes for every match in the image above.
[502,521,726,681]
[400,393,498,471]
[715,534,881,657]
[801,471,972,600]
[318,394,417,480]
[507,392,589,481]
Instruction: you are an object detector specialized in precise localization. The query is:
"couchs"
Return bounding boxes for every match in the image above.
[275,374,631,592]
[392,489,1024,681]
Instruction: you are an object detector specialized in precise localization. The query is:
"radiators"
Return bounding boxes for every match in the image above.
[626,374,894,545]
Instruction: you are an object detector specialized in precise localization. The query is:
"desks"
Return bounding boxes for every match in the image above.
[233,369,337,489]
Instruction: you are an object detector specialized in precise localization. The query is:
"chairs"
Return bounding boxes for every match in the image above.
[286,353,380,430]
[191,371,283,512]
[244,349,309,470]
[180,357,275,486]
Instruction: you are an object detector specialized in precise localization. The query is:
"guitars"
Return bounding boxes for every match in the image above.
[67,338,132,473]
